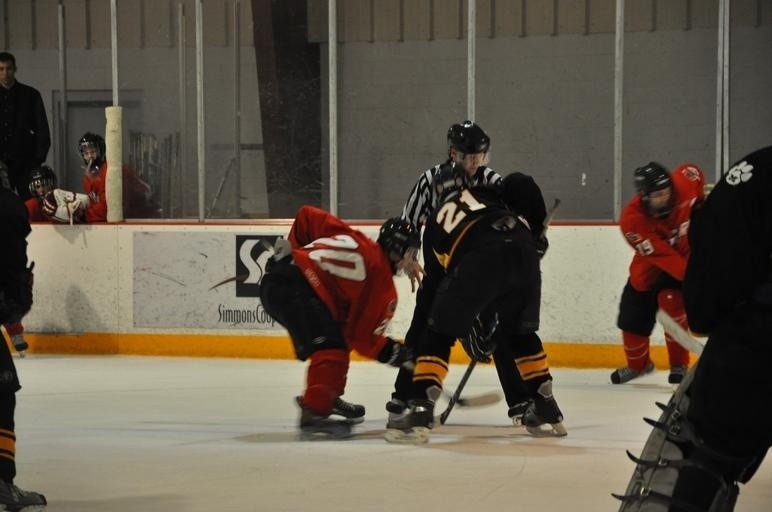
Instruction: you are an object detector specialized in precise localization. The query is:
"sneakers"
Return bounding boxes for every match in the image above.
[6,319,30,352]
[0,481,48,512]
[668,364,689,384]
[384,395,410,414]
[508,398,536,418]
[610,359,655,384]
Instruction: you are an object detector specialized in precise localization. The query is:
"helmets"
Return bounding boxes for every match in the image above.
[446,119,490,155]
[78,131,107,170]
[31,165,58,193]
[378,215,421,277]
[634,161,676,221]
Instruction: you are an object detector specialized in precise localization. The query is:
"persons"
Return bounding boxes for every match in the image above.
[608,160,706,384]
[0,51,51,198]
[610,142,772,512]
[0,185,36,353]
[259,204,422,433]
[78,132,160,223]
[1,335,49,509]
[24,165,60,223]
[387,120,533,418]
[386,171,565,426]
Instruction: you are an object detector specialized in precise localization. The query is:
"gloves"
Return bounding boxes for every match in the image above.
[534,234,549,260]
[461,322,494,363]
[263,236,295,273]
[375,336,409,368]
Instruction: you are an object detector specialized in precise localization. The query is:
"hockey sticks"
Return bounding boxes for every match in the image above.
[433,198,563,424]
[408,361,500,407]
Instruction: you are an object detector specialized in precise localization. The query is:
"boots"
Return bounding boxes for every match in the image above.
[522,377,564,428]
[294,393,353,438]
[386,385,445,431]
[331,397,365,418]
